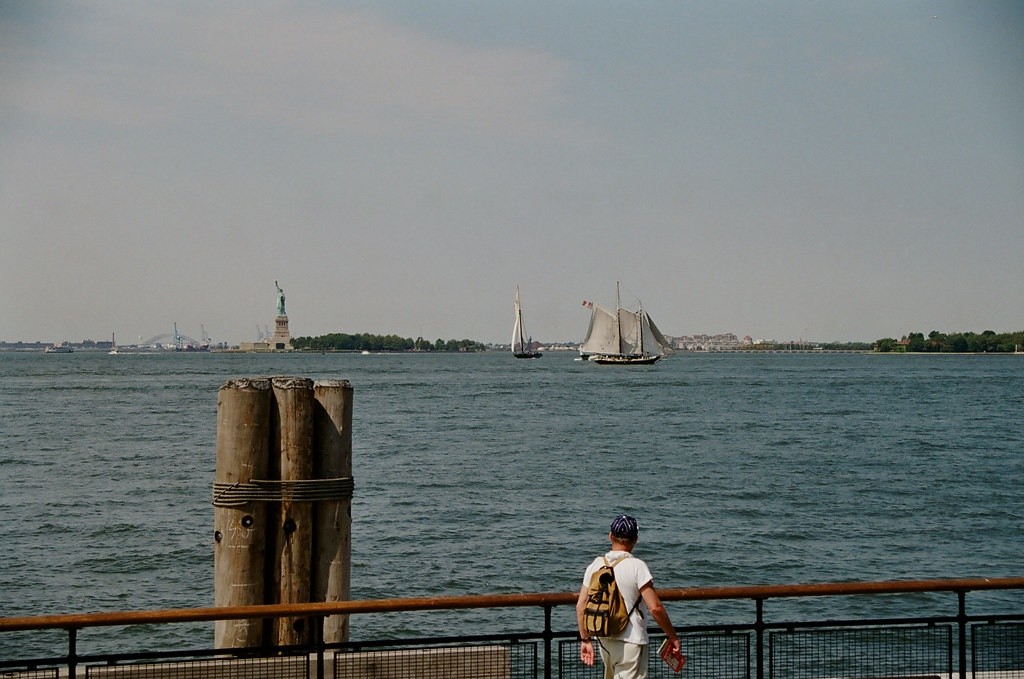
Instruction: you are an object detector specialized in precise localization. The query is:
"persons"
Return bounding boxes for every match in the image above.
[575,515,682,679]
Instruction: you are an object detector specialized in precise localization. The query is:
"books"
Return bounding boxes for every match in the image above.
[656,639,687,674]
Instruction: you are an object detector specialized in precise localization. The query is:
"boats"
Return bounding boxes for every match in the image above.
[579,282,676,365]
[44,346,75,353]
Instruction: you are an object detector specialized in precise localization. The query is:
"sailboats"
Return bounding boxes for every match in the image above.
[510,285,544,358]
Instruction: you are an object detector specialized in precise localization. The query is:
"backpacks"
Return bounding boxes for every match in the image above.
[581,556,642,638]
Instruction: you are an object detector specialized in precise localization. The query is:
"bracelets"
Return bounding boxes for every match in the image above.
[580,636,592,644]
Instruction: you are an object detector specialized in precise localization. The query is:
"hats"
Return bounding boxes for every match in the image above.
[610,515,639,538]
